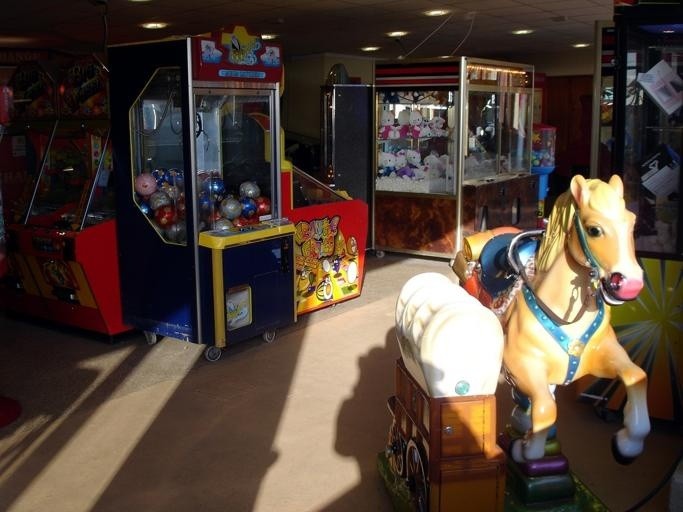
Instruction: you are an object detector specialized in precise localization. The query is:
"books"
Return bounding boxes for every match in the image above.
[636,59,683,117]
[636,143,680,201]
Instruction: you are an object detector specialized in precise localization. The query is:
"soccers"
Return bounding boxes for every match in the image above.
[134,167,270,242]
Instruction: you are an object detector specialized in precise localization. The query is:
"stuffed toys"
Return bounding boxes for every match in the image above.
[377,109,513,195]
